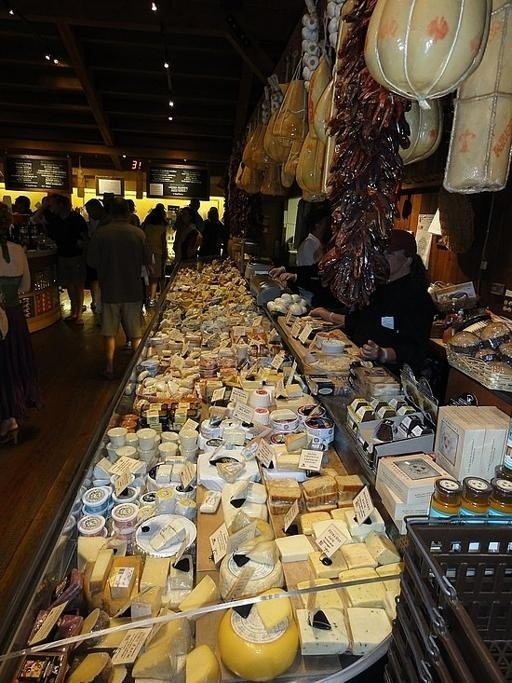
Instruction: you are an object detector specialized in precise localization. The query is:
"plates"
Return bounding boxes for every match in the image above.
[135,513,197,557]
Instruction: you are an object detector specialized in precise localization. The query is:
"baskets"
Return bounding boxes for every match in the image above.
[445,343,512,391]
[432,281,479,312]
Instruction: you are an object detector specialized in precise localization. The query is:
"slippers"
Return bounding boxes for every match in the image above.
[72,319,85,327]
[63,315,78,323]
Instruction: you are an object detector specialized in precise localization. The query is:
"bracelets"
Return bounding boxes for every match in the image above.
[329,311,336,322]
[381,347,387,364]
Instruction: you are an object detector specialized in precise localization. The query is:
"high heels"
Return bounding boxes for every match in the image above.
[0,418,20,445]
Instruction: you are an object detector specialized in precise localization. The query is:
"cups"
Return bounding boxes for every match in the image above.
[270,403,335,444]
[315,330,346,353]
[103,426,198,460]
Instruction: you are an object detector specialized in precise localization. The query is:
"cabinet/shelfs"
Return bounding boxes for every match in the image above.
[1,245,512,683]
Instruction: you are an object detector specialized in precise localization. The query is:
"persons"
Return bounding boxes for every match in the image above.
[1,203,37,446]
[127,199,225,306]
[15,196,32,213]
[85,193,150,380]
[268,256,331,307]
[36,193,104,323]
[297,209,331,280]
[308,228,435,376]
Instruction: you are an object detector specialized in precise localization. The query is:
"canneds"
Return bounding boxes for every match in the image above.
[426,423,512,525]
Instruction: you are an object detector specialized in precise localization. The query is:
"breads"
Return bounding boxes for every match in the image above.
[447,322,512,374]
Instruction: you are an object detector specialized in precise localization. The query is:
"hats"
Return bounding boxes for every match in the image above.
[377,227,418,256]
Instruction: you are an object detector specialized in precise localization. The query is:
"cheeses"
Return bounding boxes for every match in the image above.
[13,257,404,683]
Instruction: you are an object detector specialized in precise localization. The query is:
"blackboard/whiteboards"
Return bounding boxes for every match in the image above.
[146,163,210,201]
[4,154,73,194]
